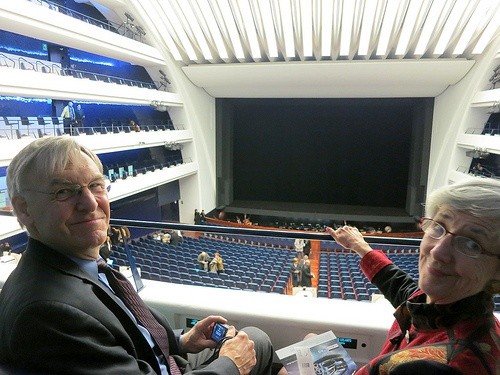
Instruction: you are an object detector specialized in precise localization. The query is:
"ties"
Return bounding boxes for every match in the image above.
[96,257,182,375]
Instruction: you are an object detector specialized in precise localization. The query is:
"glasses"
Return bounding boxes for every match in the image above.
[28,176,111,205]
[418,217,500,258]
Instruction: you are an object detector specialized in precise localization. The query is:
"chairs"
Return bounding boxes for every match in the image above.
[104,230,298,295]
[317,240,419,303]
[0,116,64,140]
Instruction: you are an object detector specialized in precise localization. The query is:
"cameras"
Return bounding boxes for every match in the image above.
[211,322,236,343]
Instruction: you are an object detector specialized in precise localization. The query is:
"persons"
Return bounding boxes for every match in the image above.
[290,255,315,288]
[303,240,311,258]
[0,135,290,375]
[0,242,13,257]
[128,120,141,132]
[215,252,224,275]
[194,208,206,225]
[75,104,86,133]
[153,229,184,246]
[99,235,113,268]
[61,101,78,135]
[197,251,211,272]
[304,177,500,375]
